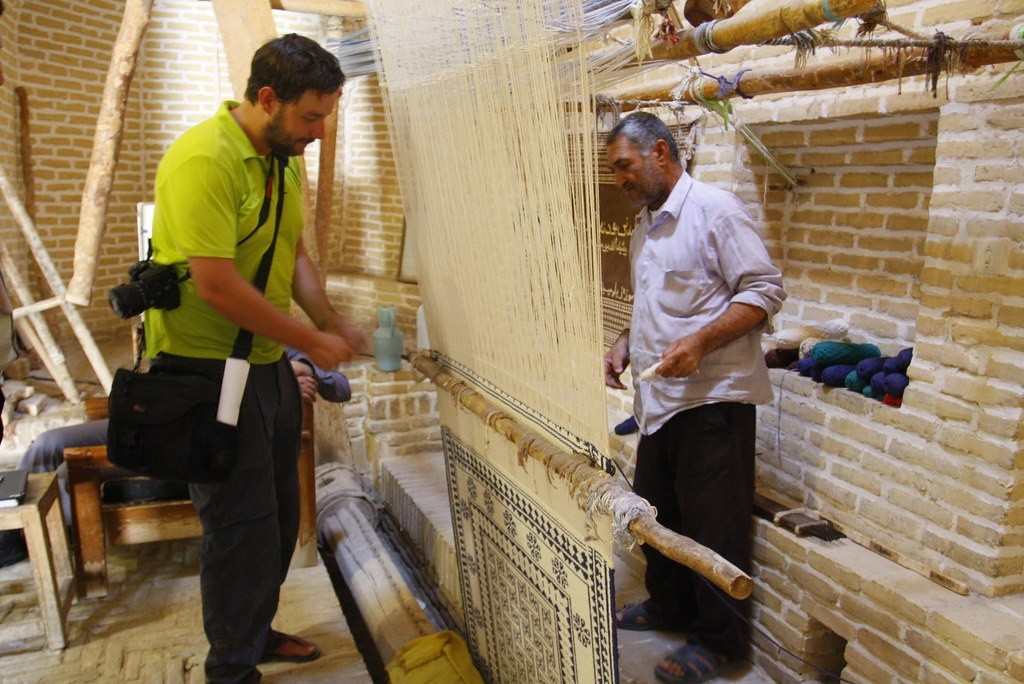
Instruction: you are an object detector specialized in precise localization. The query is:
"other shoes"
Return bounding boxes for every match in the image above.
[0,530,28,568]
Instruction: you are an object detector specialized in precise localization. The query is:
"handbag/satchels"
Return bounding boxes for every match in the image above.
[105,367,244,486]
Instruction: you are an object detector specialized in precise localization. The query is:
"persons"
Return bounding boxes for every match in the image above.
[108,30,369,679]
[600,111,790,681]
[1,351,350,532]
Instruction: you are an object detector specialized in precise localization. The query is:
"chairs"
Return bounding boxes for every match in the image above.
[64,396,320,599]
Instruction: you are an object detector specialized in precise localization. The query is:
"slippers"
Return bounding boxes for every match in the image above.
[612,600,697,633]
[253,628,322,664]
[653,642,756,684]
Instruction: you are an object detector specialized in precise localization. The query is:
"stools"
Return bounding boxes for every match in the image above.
[0,471,81,650]
